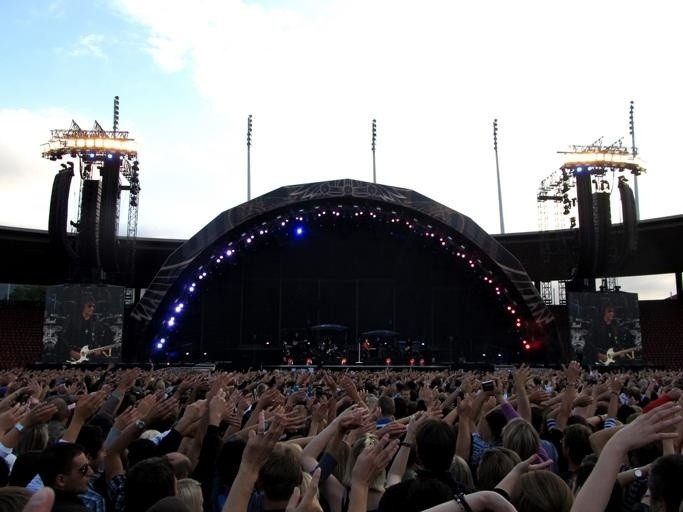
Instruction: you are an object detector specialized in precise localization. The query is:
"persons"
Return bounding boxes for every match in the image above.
[2,359,682,512]
[361,338,370,359]
[53,293,114,365]
[583,300,632,366]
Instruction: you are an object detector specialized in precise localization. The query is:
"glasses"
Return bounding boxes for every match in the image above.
[64,464,88,478]
[87,305,95,309]
[607,309,615,313]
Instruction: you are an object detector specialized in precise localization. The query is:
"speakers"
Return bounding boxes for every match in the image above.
[592,192,612,272]
[48,166,80,275]
[577,171,596,275]
[79,179,103,272]
[97,174,122,273]
[618,180,637,264]
